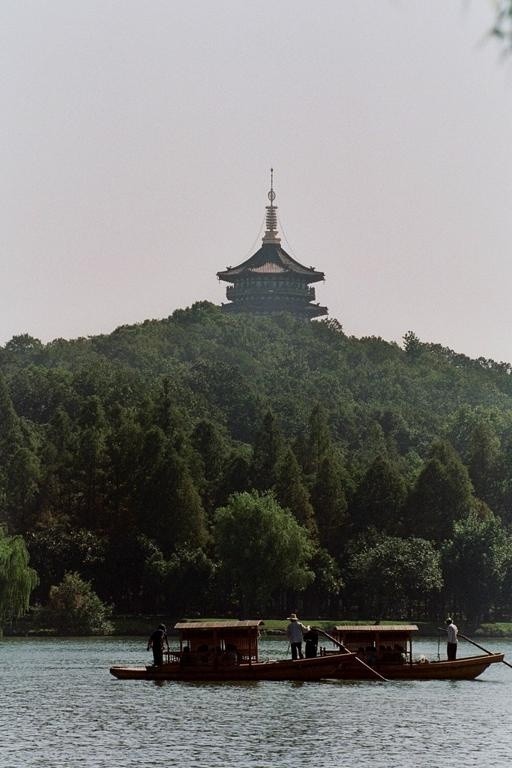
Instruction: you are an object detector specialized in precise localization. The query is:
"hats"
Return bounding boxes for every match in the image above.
[286,614,299,620]
[444,618,454,624]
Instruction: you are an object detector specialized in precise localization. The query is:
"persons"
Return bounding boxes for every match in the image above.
[357,645,408,664]
[445,617,458,661]
[180,644,240,667]
[147,624,169,665]
[287,620,318,659]
